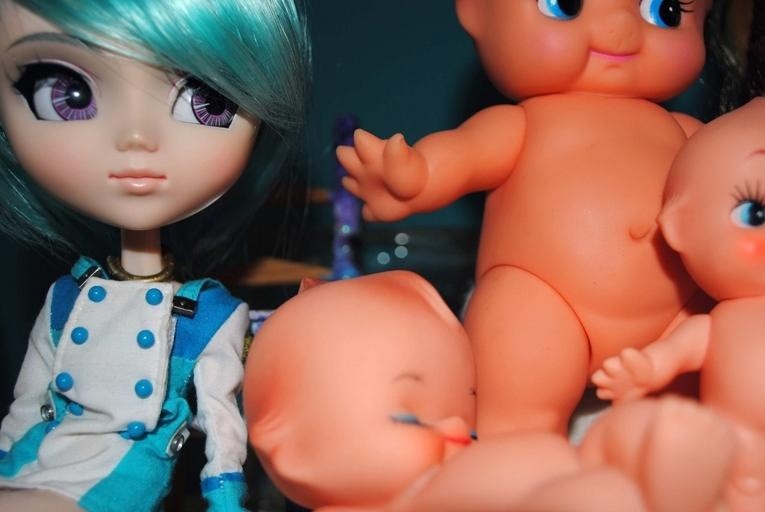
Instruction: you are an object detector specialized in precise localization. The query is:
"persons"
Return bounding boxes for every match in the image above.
[0,0,319,512]
[333,2,714,447]
[589,96,765,441]
[242,268,765,511]
